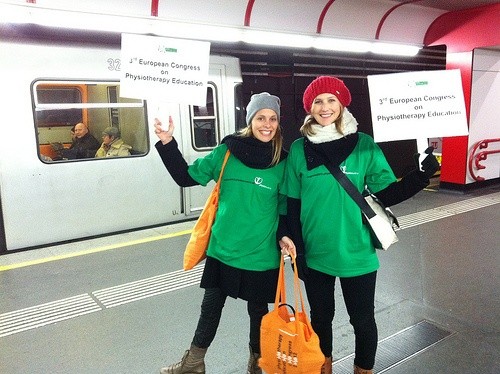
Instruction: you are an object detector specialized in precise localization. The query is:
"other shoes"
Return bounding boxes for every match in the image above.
[246,343,263,373]
[353,364,372,374]
[160,343,208,373]
[322,356,332,374]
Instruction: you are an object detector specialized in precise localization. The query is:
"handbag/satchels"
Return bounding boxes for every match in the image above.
[361,187,400,251]
[182,149,231,271]
[257,245,325,373]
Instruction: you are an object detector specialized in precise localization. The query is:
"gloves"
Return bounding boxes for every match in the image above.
[413,146,440,179]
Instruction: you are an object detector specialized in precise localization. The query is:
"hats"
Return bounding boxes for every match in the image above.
[102,127,119,137]
[304,75,351,114]
[246,92,281,126]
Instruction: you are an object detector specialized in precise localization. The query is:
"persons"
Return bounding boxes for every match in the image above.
[153,91,289,374]
[287,75,440,374]
[52,122,132,161]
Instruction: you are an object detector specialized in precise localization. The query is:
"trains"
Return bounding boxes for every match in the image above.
[0,4,447,255]
